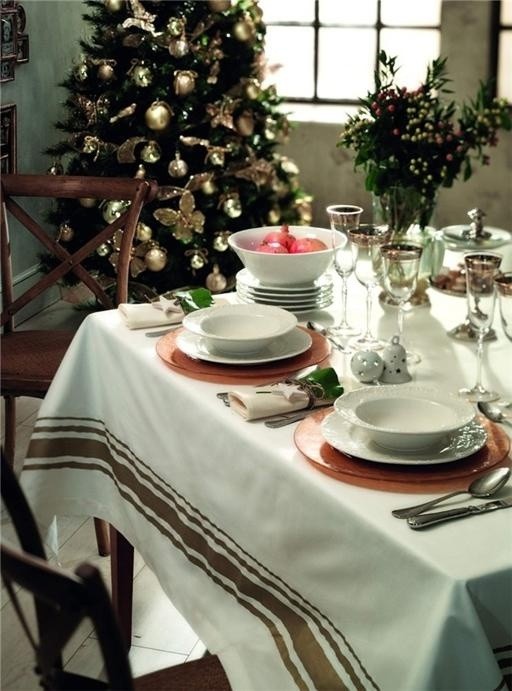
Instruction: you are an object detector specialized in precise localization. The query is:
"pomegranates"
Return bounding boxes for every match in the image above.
[289,238,326,254]
[264,224,296,250]
[255,240,288,253]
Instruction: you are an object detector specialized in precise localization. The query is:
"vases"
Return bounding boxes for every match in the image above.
[366,186,421,276]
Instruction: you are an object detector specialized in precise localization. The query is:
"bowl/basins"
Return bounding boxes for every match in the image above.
[331,385,480,452]
[225,225,347,287]
[181,303,298,357]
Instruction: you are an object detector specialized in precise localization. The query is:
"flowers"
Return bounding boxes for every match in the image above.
[340,50,511,238]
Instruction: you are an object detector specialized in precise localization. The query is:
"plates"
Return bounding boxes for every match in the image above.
[294,406,512,484]
[154,327,335,381]
[320,410,492,468]
[171,328,315,370]
[232,266,336,317]
[435,225,512,253]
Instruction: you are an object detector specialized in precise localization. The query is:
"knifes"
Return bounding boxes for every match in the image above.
[404,498,511,533]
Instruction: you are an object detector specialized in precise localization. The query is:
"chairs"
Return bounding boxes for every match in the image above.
[0,174,159,558]
[1,541,234,691]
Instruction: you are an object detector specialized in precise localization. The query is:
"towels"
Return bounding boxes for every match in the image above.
[227,369,358,424]
[118,286,211,333]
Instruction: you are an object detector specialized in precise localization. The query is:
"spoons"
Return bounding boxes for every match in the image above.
[475,401,512,430]
[390,463,511,520]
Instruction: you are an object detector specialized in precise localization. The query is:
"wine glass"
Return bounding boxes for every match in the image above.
[450,248,511,403]
[324,203,363,339]
[376,241,424,366]
[347,224,398,353]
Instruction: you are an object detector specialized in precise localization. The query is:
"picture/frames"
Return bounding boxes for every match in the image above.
[1,2,28,174]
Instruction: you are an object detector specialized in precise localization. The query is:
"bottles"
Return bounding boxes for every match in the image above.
[461,206,491,241]
